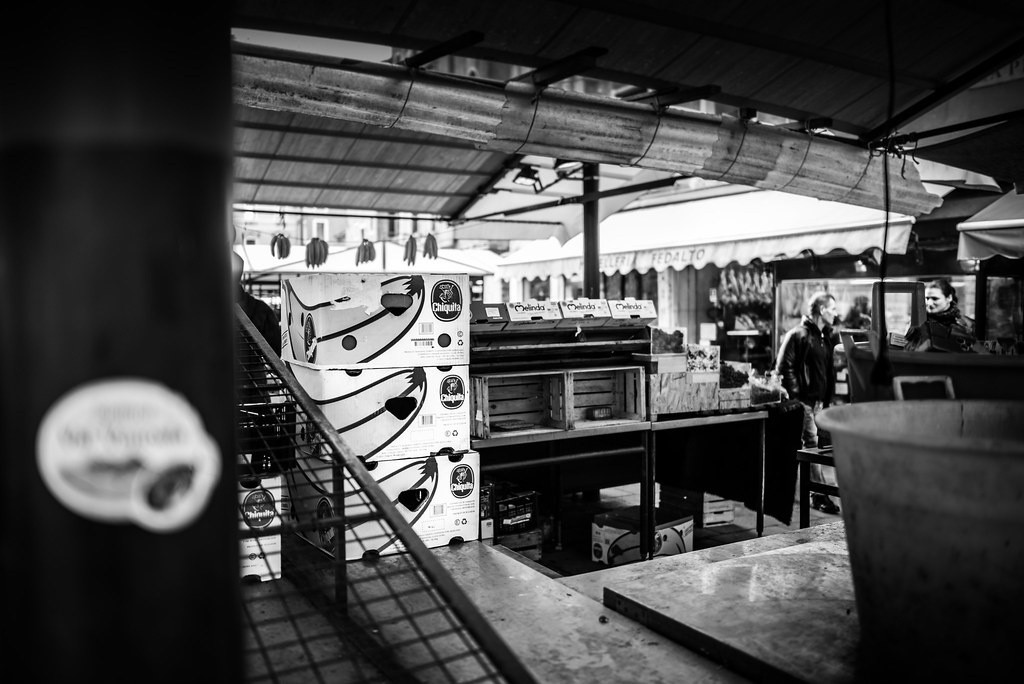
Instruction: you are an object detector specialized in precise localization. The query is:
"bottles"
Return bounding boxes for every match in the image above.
[254,450,277,479]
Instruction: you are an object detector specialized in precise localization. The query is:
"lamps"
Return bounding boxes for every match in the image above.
[513,166,543,195]
[551,158,583,174]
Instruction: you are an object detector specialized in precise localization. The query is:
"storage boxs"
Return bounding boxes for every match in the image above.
[479,481,542,563]
[471,303,510,334]
[294,449,481,561]
[238,454,286,582]
[280,272,477,371]
[285,362,470,462]
[602,297,658,330]
[555,296,611,330]
[503,298,563,331]
[569,365,647,432]
[591,505,695,566]
[470,370,570,439]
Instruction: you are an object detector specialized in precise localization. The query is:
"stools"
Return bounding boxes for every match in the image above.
[797,445,842,528]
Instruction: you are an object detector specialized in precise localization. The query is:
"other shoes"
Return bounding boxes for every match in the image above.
[811,493,840,514]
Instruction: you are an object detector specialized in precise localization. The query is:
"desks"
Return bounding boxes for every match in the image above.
[471,418,650,565]
[651,400,807,538]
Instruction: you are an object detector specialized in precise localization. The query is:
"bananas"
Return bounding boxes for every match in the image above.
[305,237,328,268]
[423,233,438,260]
[270,233,290,259]
[403,235,416,266]
[355,239,375,266]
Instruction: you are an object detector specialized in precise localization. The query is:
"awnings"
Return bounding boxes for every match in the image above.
[957,189,1024,261]
[499,173,968,284]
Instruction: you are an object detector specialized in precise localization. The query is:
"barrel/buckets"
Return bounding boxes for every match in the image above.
[816,374,1024,668]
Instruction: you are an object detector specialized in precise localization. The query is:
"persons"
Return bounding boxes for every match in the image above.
[843,295,873,342]
[775,291,849,514]
[903,279,975,354]
[233,253,281,403]
